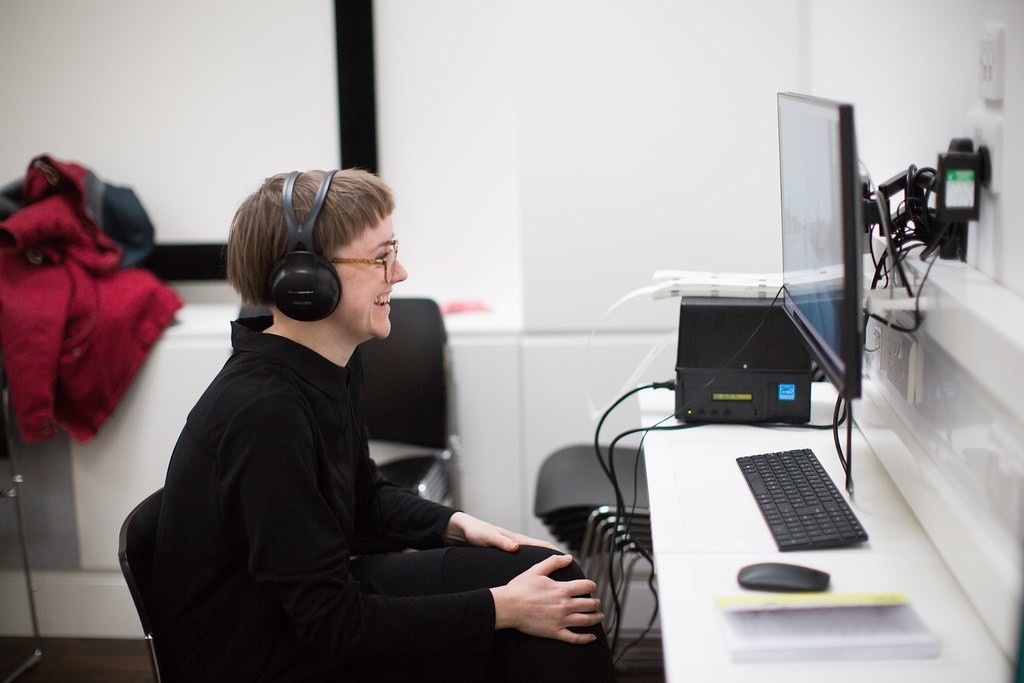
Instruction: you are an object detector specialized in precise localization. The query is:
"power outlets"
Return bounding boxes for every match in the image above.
[969,114,1002,196]
[867,316,916,403]
[978,19,1007,101]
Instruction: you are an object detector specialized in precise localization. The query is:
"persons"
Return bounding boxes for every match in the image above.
[153,169,617,683]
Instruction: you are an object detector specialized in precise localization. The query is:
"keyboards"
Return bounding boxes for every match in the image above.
[736,449,869,552]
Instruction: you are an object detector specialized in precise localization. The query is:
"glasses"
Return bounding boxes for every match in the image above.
[328,239,398,283]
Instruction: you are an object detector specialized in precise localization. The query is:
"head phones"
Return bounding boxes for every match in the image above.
[270,169,343,322]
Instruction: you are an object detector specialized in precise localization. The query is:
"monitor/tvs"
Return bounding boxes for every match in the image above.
[776,90,865,400]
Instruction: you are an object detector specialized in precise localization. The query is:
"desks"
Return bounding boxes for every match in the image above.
[635,365,1017,683]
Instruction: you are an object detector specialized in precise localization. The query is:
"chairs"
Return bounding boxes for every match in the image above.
[533,444,651,658]
[238,300,467,513]
[117,487,170,683]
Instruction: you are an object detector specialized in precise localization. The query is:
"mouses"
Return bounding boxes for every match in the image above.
[737,562,831,593]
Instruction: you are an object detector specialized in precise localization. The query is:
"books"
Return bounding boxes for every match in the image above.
[711,592,940,662]
